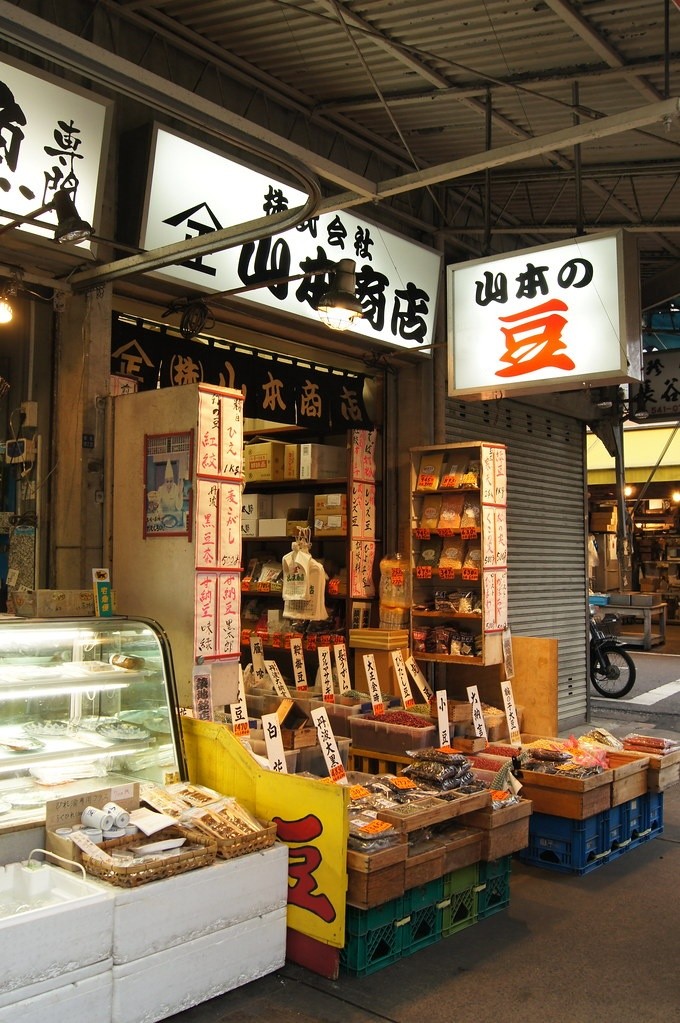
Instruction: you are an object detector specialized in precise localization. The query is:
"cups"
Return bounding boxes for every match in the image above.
[56,802,139,845]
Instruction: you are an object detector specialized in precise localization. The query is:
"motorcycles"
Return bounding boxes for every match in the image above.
[589,604,636,699]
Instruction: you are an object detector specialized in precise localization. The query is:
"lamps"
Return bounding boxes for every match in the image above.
[0,190,94,246]
[173,259,363,320]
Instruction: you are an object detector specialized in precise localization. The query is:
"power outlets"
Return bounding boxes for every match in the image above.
[19,401,38,429]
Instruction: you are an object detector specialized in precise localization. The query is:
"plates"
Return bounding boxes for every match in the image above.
[22,720,80,738]
[96,722,151,741]
[0,792,60,816]
[128,837,186,855]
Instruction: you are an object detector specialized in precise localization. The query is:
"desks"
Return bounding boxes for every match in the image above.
[600,603,668,651]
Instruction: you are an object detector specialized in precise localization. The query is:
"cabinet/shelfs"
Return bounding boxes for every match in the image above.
[618,534,680,623]
[408,441,508,667]
[241,421,382,672]
[0,620,188,863]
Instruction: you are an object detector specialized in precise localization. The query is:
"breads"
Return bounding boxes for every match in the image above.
[112,654,138,670]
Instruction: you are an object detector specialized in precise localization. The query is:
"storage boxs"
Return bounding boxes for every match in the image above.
[272,493,314,519]
[258,518,286,536]
[11,590,117,615]
[314,494,347,515]
[315,515,347,536]
[286,506,313,536]
[589,596,609,605]
[631,594,661,606]
[242,493,272,520]
[284,444,300,480]
[638,575,660,592]
[244,436,289,482]
[609,594,631,606]
[242,520,258,537]
[0,686,680,1023]
[300,444,347,481]
